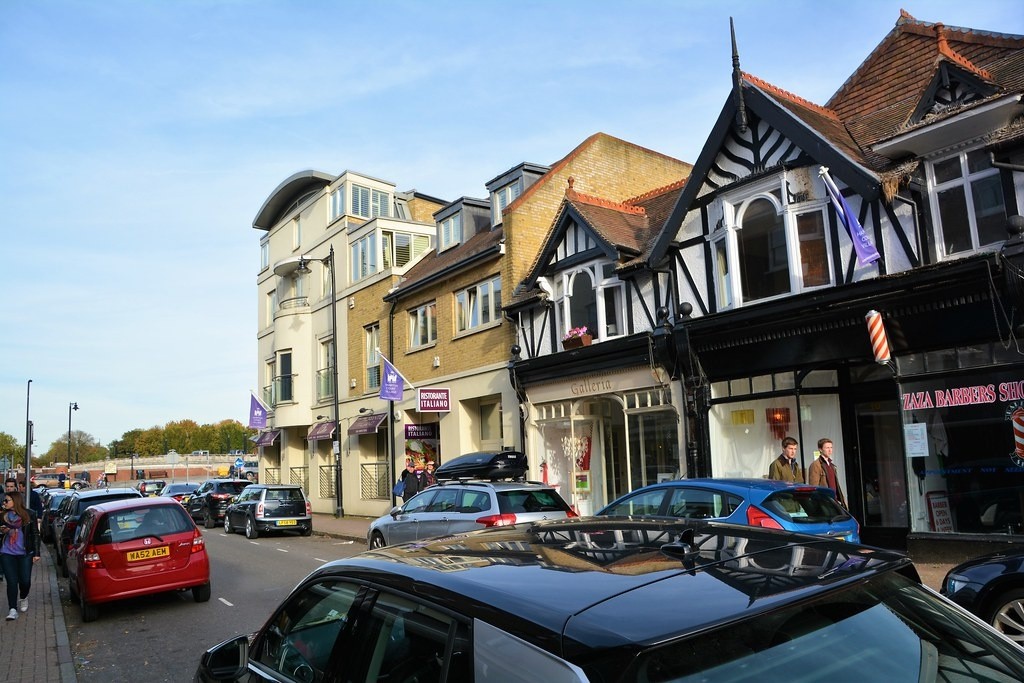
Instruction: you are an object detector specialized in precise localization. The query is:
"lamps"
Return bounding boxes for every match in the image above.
[317,415,329,419]
[731,402,754,425]
[360,408,373,413]
[800,395,812,420]
[560,433,588,466]
[766,398,790,441]
[865,309,891,366]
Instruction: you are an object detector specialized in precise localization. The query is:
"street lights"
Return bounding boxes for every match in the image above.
[295,243,346,519]
[67,402,80,481]
[25,379,35,509]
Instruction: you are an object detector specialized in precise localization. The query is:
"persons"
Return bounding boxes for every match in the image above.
[418,461,443,492]
[58,480,61,488]
[58,471,65,488]
[134,512,168,537]
[809,438,848,518]
[401,461,420,504]
[97,473,107,487]
[83,477,87,481]
[769,437,804,513]
[140,470,146,479]
[0,478,44,619]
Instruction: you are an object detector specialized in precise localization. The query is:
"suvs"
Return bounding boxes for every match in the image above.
[30,473,87,491]
[49,487,143,565]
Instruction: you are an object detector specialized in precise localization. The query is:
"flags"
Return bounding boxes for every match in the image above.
[380,360,404,401]
[248,393,267,429]
[820,176,881,266]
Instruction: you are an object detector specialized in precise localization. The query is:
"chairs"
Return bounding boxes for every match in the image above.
[144,512,168,533]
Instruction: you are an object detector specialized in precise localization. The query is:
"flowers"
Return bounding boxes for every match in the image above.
[563,325,587,340]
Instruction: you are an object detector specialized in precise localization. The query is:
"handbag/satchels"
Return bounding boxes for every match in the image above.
[393,474,409,497]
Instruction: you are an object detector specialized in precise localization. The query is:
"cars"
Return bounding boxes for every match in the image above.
[578,476,862,581]
[66,497,211,622]
[157,484,201,503]
[32,487,84,545]
[936,542,1024,648]
[191,450,208,454]
[186,478,254,530]
[134,479,169,498]
[365,449,580,553]
[222,484,313,539]
[193,515,1023,683]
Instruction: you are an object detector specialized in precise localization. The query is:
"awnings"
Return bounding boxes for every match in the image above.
[308,422,341,441]
[255,431,281,446]
[347,414,387,436]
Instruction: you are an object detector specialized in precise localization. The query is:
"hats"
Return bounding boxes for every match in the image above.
[427,461,435,464]
[406,463,415,467]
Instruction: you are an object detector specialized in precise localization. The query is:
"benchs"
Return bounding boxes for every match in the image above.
[149,471,168,479]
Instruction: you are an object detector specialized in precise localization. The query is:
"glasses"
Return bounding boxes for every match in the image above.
[2,498,12,504]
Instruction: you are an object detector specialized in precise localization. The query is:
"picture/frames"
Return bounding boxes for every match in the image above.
[572,470,592,494]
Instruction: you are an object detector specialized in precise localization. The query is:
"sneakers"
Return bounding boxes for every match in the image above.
[5,608,18,620]
[20,597,29,612]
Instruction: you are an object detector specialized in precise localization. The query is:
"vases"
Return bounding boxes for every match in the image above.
[562,335,592,350]
